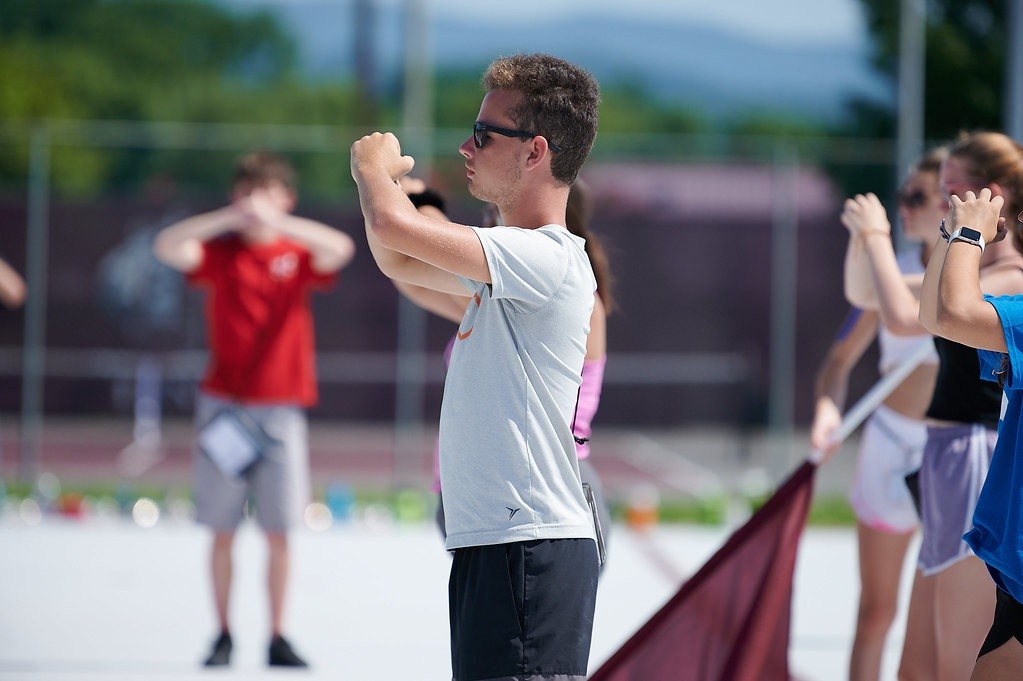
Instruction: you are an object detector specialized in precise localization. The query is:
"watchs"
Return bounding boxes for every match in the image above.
[948,226,986,254]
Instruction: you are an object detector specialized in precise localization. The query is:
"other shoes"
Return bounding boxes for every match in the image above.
[206,633,232,666]
[269,637,306,667]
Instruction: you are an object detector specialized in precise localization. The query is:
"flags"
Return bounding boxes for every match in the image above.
[587,458,819,681]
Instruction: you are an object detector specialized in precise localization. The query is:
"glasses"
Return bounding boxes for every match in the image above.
[470,121,562,154]
[896,192,945,207]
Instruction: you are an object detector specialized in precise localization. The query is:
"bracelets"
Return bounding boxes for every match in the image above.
[859,228,893,243]
[407,188,449,214]
[939,218,950,243]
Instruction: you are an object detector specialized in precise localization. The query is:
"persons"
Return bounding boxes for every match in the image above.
[396,176,612,556]
[154,152,355,668]
[350,51,600,681]
[809,149,948,681]
[839,132,1023,681]
[918,188,1023,681]
